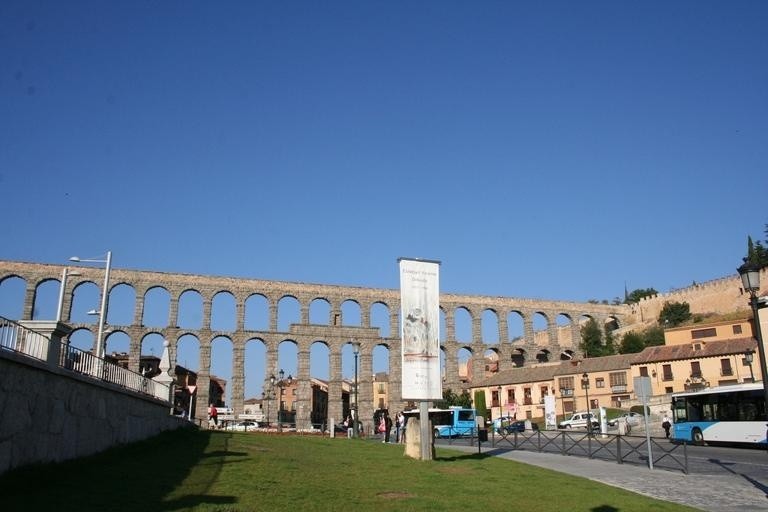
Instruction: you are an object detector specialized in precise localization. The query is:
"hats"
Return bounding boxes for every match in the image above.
[608,411,644,427]
[557,412,601,432]
[219,420,259,432]
[493,416,539,434]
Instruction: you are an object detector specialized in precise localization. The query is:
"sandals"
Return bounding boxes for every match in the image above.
[398,408,476,439]
[670,383,768,448]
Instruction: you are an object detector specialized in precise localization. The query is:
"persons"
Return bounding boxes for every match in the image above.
[662,414,672,437]
[344,410,408,444]
[209,403,218,424]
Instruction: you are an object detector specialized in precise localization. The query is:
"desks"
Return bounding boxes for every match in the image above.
[581,372,590,433]
[496,385,502,434]
[54,250,113,378]
[270,368,292,433]
[352,340,361,440]
